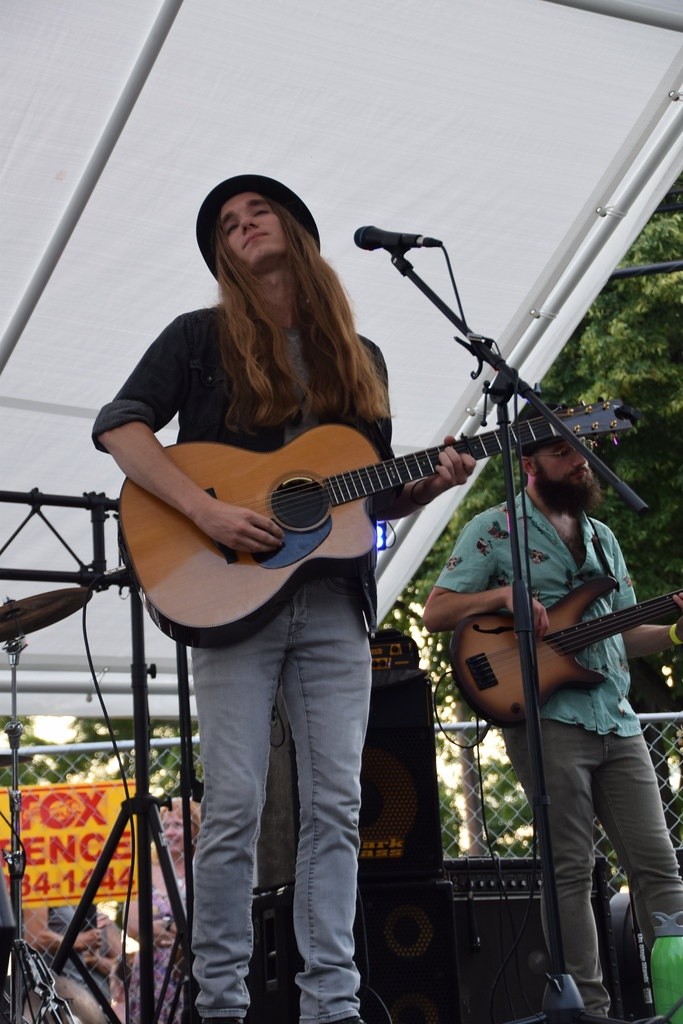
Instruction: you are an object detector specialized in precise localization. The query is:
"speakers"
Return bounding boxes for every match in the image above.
[452,894,625,1024]
[254,677,446,892]
[243,879,464,1024]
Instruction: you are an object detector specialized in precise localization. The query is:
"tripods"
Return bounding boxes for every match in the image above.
[0,628,74,1024]
[386,248,650,1024]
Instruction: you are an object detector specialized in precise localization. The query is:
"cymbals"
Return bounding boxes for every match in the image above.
[0,586,93,644]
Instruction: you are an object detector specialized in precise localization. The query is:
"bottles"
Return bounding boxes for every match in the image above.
[646,910,683,1024]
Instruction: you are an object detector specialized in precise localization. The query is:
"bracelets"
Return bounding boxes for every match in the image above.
[411,479,431,505]
[670,623,682,645]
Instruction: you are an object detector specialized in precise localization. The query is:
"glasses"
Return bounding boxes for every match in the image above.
[532,438,596,461]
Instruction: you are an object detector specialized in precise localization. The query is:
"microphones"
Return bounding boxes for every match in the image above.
[354,225,443,251]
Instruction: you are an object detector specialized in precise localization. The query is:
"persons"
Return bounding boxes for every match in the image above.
[120,798,203,1024]
[422,402,683,1023]
[23,901,136,1024]
[92,175,477,1024]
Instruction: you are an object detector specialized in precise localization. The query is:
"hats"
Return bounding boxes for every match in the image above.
[195,175,320,282]
[518,404,572,458]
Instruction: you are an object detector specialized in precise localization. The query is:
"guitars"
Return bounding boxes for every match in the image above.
[448,574,683,729]
[114,390,641,652]
